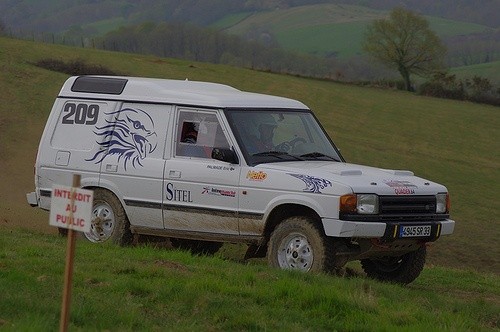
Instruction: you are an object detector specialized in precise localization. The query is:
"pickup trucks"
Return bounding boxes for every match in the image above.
[27,75,455,285]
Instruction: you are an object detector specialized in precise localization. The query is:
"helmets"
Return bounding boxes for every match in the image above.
[254,113,278,138]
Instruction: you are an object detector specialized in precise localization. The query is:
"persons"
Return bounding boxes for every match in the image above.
[248,115,291,152]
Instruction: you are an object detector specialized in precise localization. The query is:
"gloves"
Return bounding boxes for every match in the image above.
[276,142,293,155]
